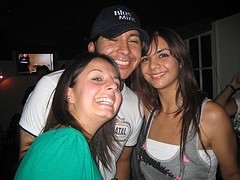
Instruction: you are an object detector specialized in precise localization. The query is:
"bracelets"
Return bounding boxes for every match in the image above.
[226,84,235,93]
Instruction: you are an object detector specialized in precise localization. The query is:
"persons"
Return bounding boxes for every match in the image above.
[13,51,240,180]
[18,5,143,180]
[131,25,240,180]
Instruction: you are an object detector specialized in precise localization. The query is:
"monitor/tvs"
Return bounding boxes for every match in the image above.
[15,51,56,75]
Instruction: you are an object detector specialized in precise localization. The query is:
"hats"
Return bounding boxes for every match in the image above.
[91,5,149,45]
